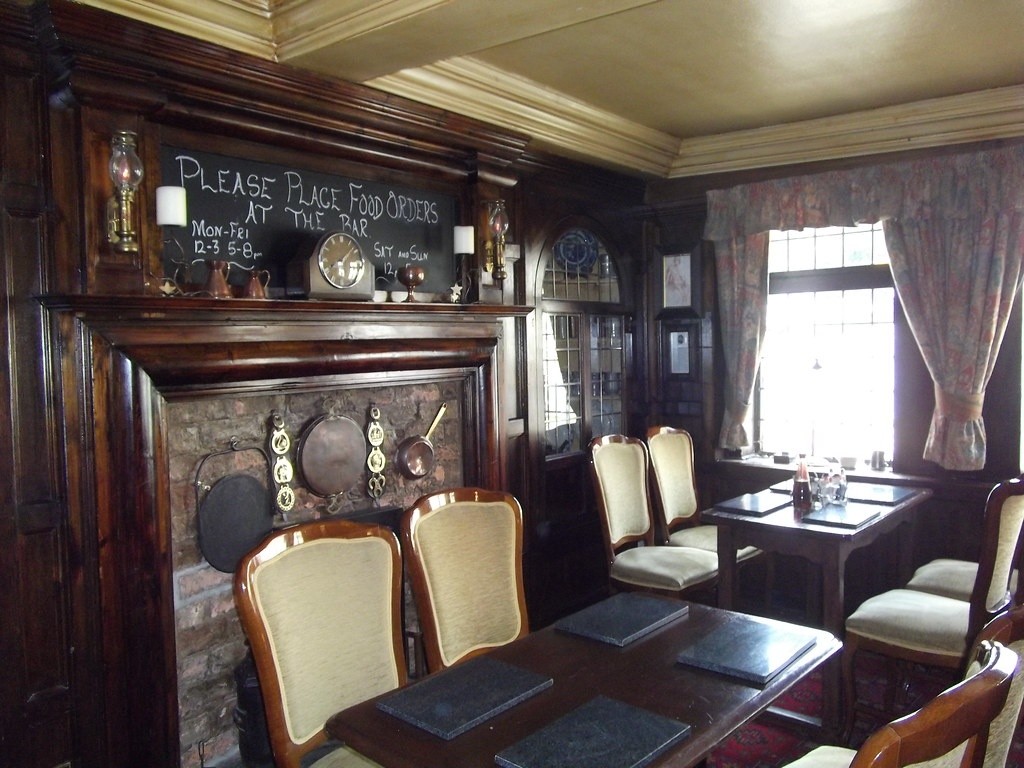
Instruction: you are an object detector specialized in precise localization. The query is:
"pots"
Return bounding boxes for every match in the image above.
[396,402,447,480]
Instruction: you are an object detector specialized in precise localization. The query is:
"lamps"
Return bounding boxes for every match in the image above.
[106,128,145,254]
[483,198,510,279]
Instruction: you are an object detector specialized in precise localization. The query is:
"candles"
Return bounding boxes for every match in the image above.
[156,185,187,227]
[454,226,474,254]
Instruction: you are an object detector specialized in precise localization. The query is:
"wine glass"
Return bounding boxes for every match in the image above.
[397,266,424,302]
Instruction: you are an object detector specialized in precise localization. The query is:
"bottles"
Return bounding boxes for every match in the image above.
[793,454,812,509]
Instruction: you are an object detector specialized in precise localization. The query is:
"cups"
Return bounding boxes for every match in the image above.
[841,457,855,468]
[871,451,884,469]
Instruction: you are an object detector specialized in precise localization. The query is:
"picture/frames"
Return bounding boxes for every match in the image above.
[652,240,702,321]
[662,322,700,383]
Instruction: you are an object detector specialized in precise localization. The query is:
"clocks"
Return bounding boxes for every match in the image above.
[317,233,365,289]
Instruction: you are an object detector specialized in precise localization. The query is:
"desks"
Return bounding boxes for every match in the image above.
[698,468,933,747]
[322,594,842,768]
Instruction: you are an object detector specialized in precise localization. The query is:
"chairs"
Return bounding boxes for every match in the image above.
[236,424,1024,768]
